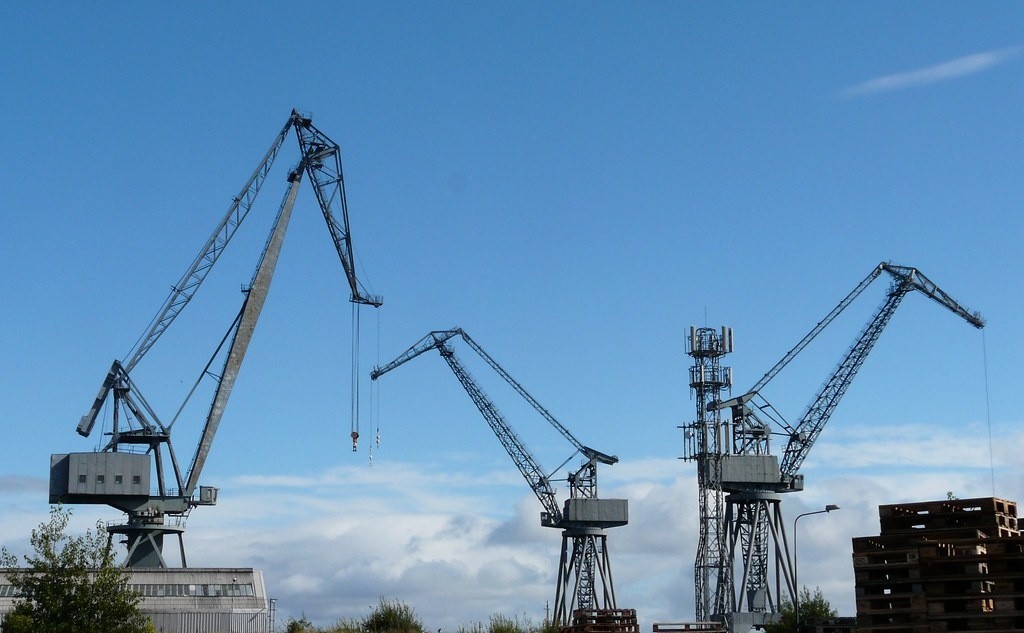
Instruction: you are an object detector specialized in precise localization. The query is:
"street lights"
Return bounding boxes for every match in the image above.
[794,504,842,633]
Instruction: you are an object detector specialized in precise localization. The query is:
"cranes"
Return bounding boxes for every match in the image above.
[366,327,640,632]
[44,111,386,565]
[673,258,988,633]
